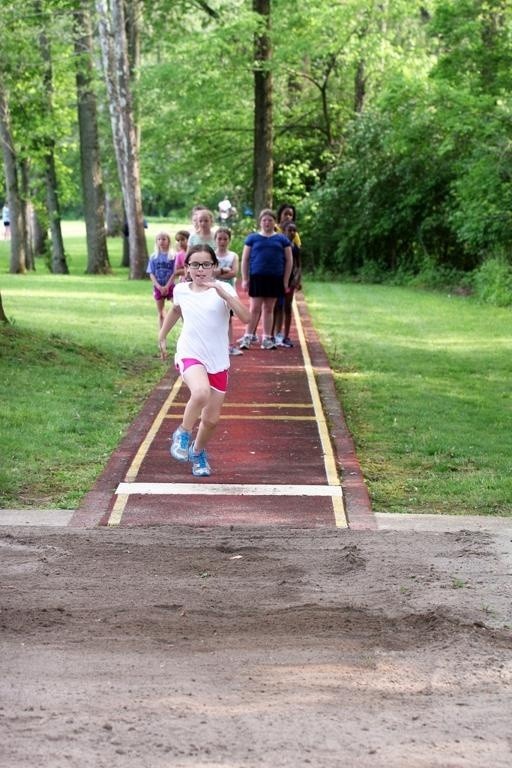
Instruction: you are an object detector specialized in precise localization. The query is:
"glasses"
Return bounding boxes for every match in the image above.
[185,260,216,269]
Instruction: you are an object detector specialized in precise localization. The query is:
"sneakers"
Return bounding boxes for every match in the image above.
[229,347,243,356]
[170,424,192,465]
[189,440,212,476]
[261,332,293,350]
[237,334,258,349]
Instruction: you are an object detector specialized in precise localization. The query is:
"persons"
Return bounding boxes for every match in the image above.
[271,221,303,349]
[2,202,12,242]
[157,244,254,479]
[211,227,244,356]
[146,230,176,364]
[172,230,191,282]
[238,206,294,351]
[273,204,302,248]
[219,194,234,229]
[183,207,217,283]
[191,204,206,230]
